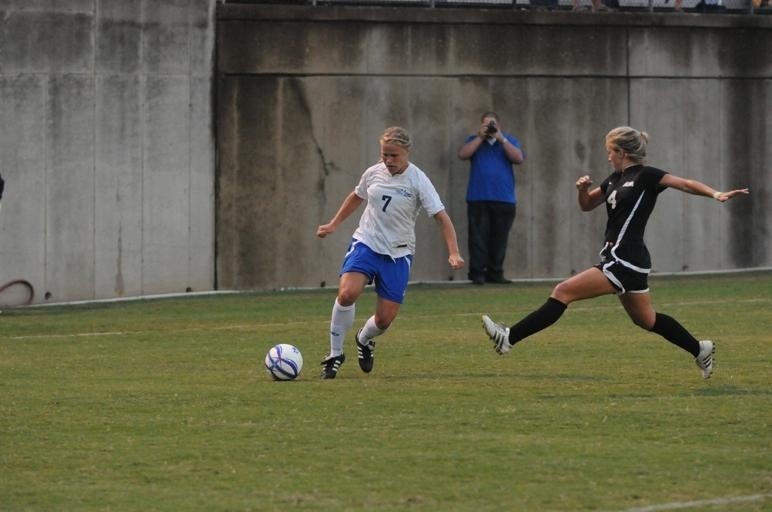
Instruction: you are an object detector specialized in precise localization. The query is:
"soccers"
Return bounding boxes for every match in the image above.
[265,342,304,382]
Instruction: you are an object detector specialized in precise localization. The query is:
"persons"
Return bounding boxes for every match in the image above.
[458,113,524,285]
[480,125,749,379]
[317,127,464,380]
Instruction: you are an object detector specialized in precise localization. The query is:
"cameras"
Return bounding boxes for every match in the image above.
[484,124,496,133]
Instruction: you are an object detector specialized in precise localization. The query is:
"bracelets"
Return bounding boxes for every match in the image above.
[499,137,508,145]
[713,191,723,200]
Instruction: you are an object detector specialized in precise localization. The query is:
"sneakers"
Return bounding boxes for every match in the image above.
[319,352,345,379]
[693,340,715,379]
[472,273,487,286]
[485,273,512,283]
[355,328,375,373]
[481,314,513,355]
